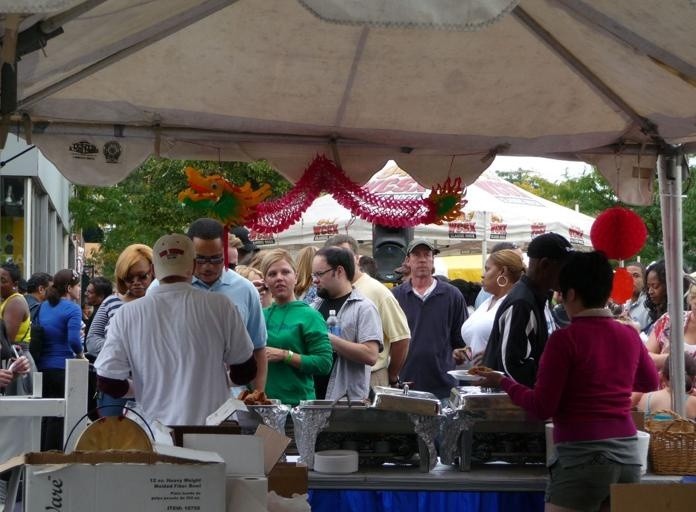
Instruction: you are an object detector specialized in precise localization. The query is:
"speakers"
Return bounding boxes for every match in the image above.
[372,221,413,283]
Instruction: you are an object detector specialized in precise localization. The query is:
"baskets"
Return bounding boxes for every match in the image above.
[643,408,696,476]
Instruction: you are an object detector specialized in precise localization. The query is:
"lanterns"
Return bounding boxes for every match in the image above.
[589,205,648,304]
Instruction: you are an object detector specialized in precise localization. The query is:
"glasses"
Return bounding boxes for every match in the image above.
[68,270,80,284]
[123,268,151,282]
[194,255,224,264]
[310,266,337,279]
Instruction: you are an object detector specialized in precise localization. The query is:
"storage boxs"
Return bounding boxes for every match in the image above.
[0,397,308,512]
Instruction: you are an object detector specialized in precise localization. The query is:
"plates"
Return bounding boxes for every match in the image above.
[448,368,503,382]
[313,450,361,476]
[238,398,282,406]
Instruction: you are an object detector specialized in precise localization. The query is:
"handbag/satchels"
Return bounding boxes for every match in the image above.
[28,301,44,366]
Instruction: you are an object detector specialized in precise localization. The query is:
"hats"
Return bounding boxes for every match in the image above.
[406,238,433,256]
[527,232,575,260]
[152,233,197,281]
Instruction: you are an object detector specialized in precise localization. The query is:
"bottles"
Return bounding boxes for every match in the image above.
[327,310,341,353]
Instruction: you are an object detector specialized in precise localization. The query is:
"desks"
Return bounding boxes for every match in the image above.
[285,454,684,511]
